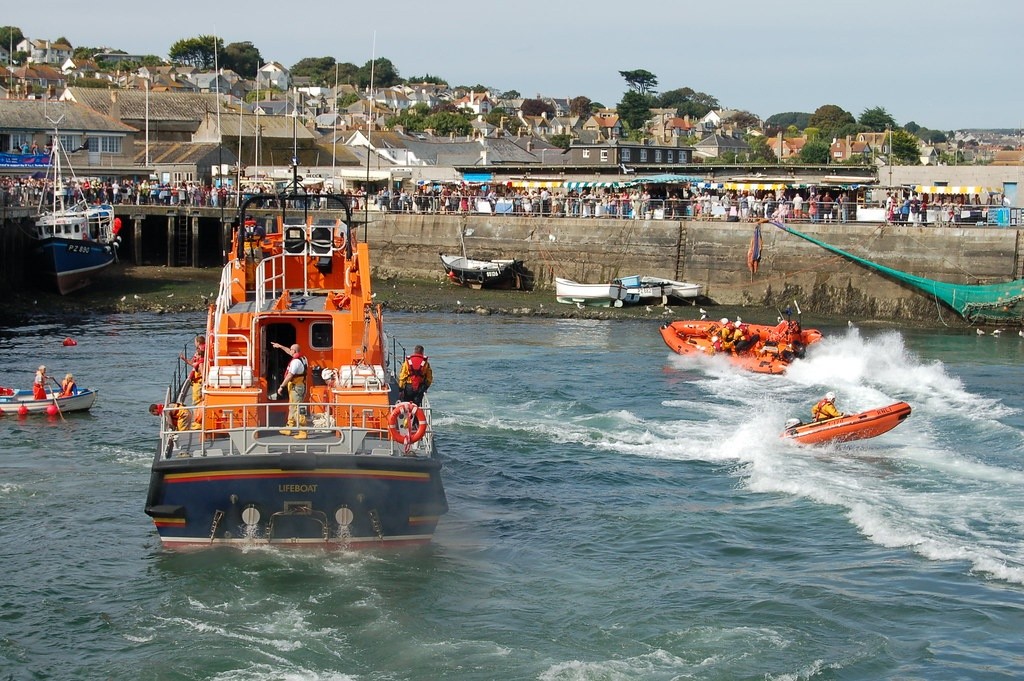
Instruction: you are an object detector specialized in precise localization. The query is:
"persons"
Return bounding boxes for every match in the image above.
[271,342,308,439]
[149,403,192,431]
[0,176,367,210]
[377,186,1010,227]
[180,336,206,430]
[57,373,78,398]
[811,392,844,423]
[33,365,50,400]
[398,345,433,407]
[20,140,50,155]
[708,318,750,356]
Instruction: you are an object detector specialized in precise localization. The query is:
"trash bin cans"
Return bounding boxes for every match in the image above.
[645,210,653,220]
[1010,207,1022,227]
[595,202,605,218]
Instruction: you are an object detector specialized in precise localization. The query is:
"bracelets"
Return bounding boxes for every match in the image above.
[280,385,284,388]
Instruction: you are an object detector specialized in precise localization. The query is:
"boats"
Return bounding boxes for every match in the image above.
[438,218,525,290]
[611,274,704,308]
[768,220,1024,327]
[779,401,912,448]
[554,277,628,308]
[142,32,452,556]
[658,317,823,376]
[0,385,100,413]
[28,114,122,296]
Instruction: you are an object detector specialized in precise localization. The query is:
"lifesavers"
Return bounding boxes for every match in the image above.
[460,275,464,282]
[477,275,486,284]
[388,404,427,443]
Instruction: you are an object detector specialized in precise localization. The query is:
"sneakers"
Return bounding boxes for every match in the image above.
[191,422,201,430]
[279,430,291,436]
[293,433,307,439]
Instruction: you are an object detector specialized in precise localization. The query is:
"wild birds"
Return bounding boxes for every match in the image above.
[371,292,377,299]
[576,302,585,309]
[848,321,855,329]
[134,294,141,302]
[1019,330,1024,338]
[778,317,782,323]
[977,329,985,336]
[662,306,676,315]
[34,301,38,304]
[699,307,707,314]
[994,330,1002,336]
[737,316,742,321]
[457,300,461,305]
[167,293,174,298]
[540,304,545,309]
[645,306,653,313]
[121,296,127,302]
[210,292,213,297]
[701,314,710,320]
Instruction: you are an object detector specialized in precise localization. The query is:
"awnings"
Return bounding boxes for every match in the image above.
[512,173,723,190]
[724,182,1002,194]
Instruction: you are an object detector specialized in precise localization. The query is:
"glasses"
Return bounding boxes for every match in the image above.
[290,348,294,351]
